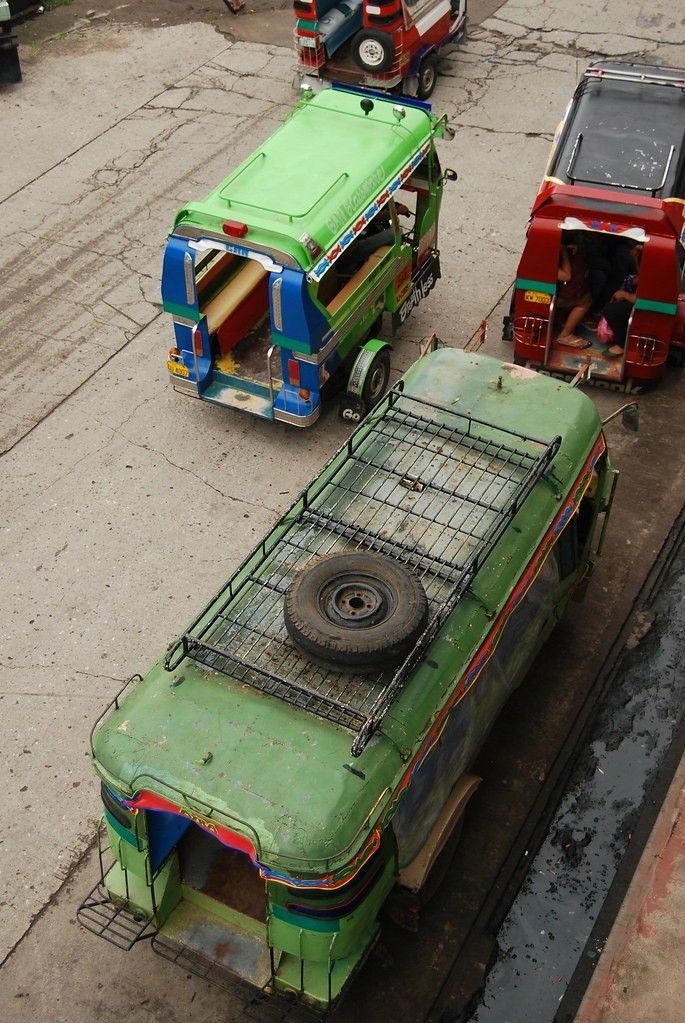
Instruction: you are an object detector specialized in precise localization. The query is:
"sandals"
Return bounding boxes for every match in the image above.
[602,345,624,358]
[557,333,591,349]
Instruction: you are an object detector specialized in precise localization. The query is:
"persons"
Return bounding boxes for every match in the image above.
[351,202,408,267]
[558,232,644,356]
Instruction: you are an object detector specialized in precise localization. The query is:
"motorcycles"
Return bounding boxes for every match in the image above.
[501,59,685,396]
[292,0,470,100]
[161,86,457,430]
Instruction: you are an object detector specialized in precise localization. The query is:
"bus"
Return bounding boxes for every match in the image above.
[80,333,640,1017]
